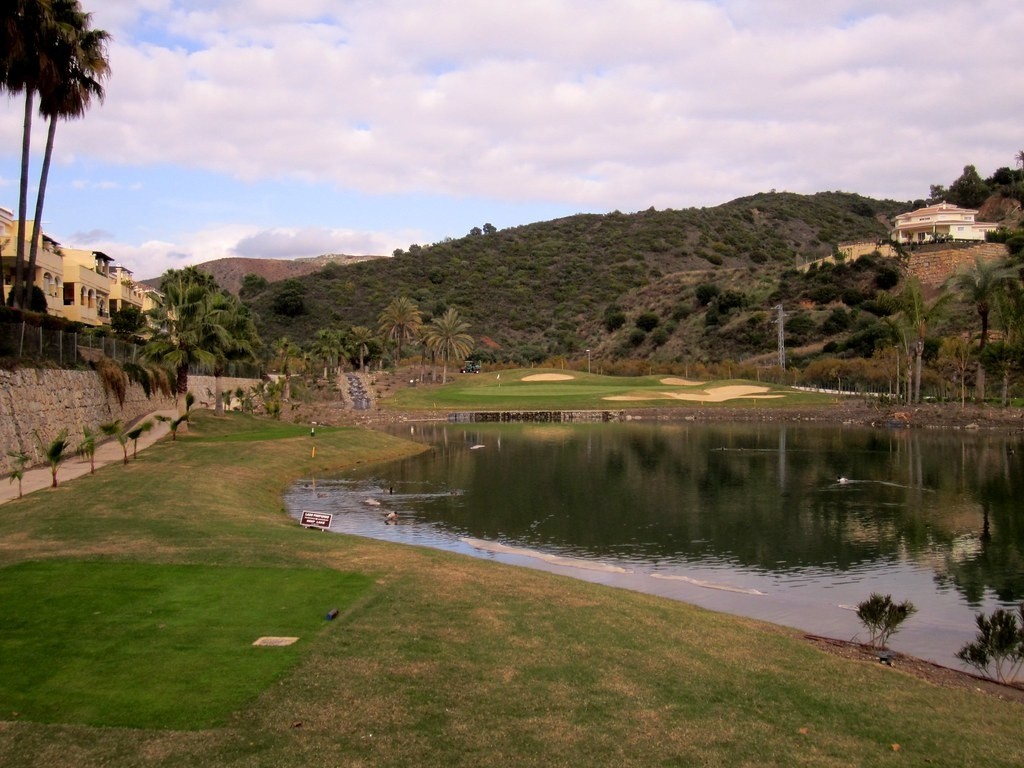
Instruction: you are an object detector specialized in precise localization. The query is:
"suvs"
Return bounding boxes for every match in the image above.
[461,360,480,374]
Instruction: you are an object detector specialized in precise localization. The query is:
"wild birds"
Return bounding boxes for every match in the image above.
[836,476,848,484]
[380,511,400,520]
